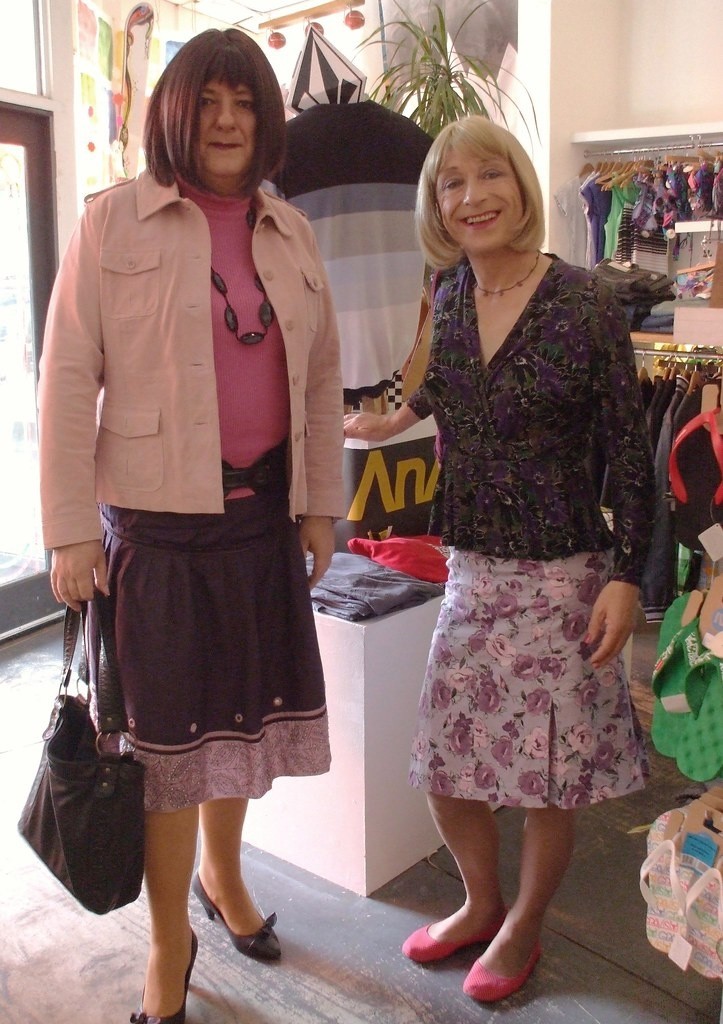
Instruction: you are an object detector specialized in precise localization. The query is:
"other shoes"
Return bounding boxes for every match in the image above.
[402,900,508,961]
[463,940,544,1000]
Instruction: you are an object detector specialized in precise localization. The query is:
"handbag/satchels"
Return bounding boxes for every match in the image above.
[17,588,146,915]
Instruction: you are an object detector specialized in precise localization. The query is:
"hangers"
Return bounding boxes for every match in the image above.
[563,141,723,193]
[638,350,723,421]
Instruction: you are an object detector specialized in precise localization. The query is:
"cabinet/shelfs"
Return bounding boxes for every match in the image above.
[198,550,505,898]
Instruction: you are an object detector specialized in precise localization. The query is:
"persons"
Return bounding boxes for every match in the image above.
[344,114,657,1000]
[38,30,345,1024]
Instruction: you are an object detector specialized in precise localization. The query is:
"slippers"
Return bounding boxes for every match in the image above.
[668,379,723,552]
[651,589,723,783]
[640,807,723,980]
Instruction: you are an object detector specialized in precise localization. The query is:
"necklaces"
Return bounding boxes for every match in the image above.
[211,199,274,344]
[477,247,541,296]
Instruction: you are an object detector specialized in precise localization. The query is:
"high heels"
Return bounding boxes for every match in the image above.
[129,925,198,1024]
[192,869,281,961]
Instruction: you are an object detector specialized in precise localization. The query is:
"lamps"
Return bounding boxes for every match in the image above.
[257,0,365,49]
